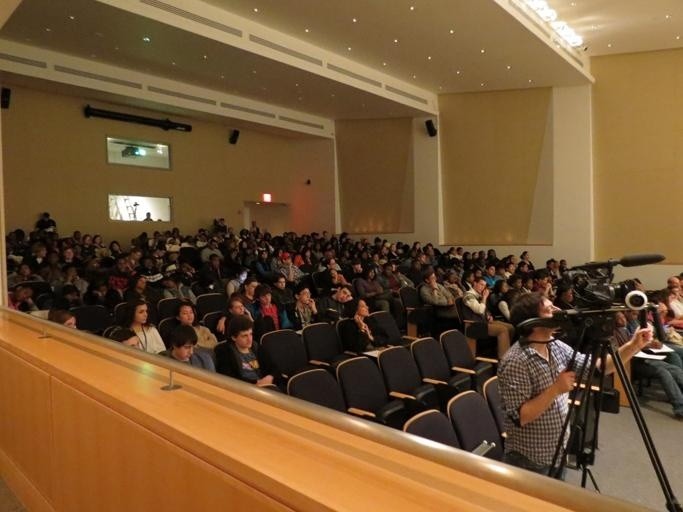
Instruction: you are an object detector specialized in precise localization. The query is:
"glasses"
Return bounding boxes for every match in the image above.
[299,291,311,295]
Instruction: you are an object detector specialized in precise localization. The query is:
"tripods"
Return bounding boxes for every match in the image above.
[547,334,683,512]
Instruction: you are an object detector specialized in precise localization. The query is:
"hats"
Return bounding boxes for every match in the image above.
[280,252,291,259]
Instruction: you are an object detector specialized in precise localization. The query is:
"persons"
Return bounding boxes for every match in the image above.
[5,209,682,420]
[496,291,655,481]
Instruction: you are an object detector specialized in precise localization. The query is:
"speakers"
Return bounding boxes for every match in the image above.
[424,119,436,137]
[0,87,11,109]
[227,128,241,144]
